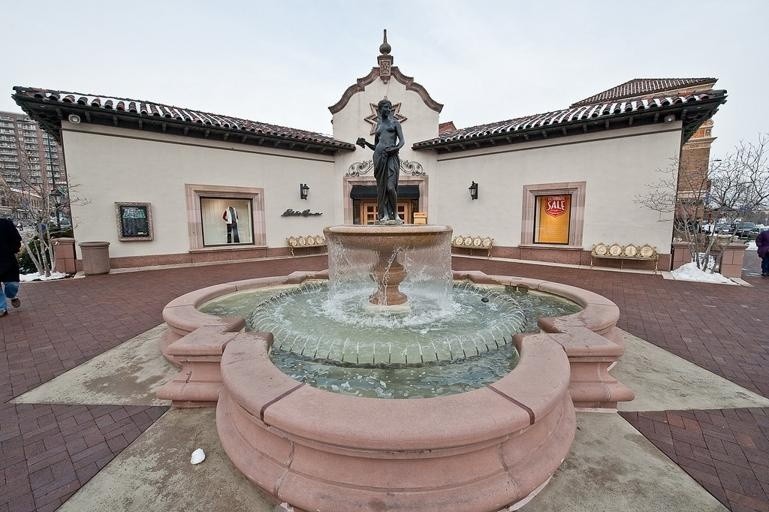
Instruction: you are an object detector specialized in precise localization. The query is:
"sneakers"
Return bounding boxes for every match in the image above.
[11,297,21,308]
[0,309,9,318]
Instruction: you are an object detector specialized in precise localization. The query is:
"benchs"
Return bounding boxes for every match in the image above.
[287,234,328,258]
[590,244,660,275]
[451,235,494,259]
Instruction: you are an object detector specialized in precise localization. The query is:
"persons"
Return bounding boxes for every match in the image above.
[355,99,405,225]
[755,228,769,277]
[0,217,22,318]
[223,206,240,243]
[16,218,23,231]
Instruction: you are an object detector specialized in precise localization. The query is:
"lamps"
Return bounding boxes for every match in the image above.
[468,180,478,200]
[300,184,310,200]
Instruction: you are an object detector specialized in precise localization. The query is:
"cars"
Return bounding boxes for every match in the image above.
[701,216,769,238]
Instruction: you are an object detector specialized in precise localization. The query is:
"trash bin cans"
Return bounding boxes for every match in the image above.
[53,238,75,274]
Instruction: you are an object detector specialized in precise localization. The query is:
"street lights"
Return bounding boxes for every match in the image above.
[50,189,63,232]
